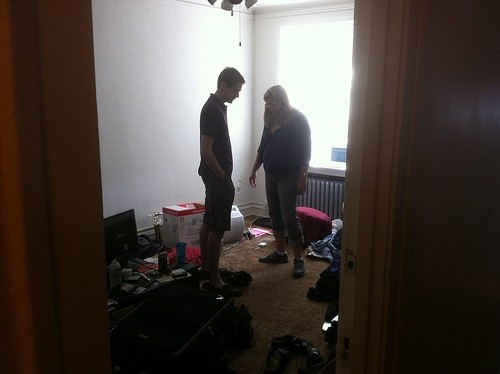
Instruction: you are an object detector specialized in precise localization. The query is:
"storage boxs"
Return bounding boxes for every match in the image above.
[162,202,207,245]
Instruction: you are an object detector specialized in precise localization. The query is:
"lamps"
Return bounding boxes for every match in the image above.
[204,0,261,46]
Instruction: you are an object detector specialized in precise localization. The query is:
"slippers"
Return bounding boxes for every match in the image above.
[271,336,315,354]
[264,346,289,374]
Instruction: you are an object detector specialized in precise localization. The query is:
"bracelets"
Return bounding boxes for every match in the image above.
[301,171,307,176]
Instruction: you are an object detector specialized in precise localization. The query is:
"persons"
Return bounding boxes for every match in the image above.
[199,68,244,298]
[249,86,310,279]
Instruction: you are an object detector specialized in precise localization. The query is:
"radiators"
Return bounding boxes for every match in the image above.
[297,178,344,219]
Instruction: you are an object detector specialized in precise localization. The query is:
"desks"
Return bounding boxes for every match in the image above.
[107,247,199,310]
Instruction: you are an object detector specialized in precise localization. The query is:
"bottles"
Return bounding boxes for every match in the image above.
[109,258,122,294]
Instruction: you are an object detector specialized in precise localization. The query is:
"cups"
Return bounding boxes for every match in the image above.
[158,254,172,274]
[176,241,187,263]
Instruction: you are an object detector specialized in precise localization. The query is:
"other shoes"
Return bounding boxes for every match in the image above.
[259,251,290,263]
[306,347,325,371]
[293,258,305,277]
[207,281,242,296]
[198,267,223,279]
